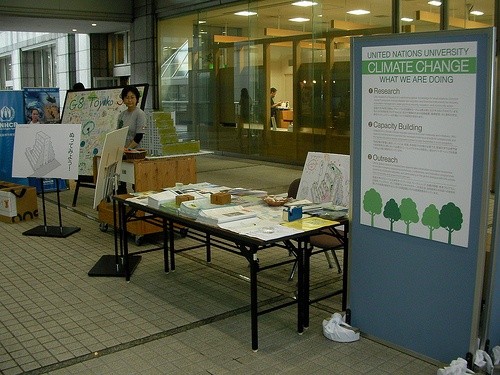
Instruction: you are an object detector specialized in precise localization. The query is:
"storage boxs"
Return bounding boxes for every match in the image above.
[0,181,39,224]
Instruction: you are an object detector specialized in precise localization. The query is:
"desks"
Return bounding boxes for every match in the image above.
[112,181,350,353]
[275,108,293,129]
[93,148,214,247]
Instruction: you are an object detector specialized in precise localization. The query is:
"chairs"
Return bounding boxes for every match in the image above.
[281,177,346,281]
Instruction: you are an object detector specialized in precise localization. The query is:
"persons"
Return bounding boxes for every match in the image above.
[237,88,252,139]
[270,88,280,117]
[30,109,40,124]
[116,86,146,195]
